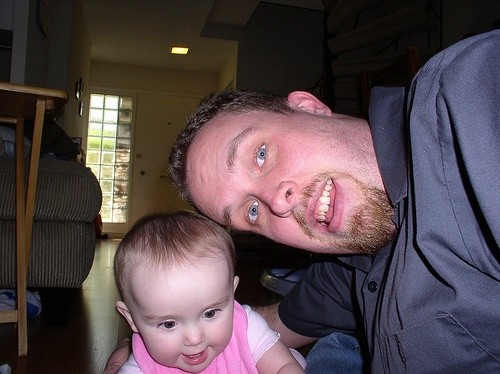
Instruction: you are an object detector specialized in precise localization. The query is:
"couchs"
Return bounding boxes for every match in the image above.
[0,125,103,289]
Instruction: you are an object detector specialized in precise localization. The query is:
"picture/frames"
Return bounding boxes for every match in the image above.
[38,0,50,41]
[75,77,85,117]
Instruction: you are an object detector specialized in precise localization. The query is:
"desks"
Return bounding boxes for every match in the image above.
[0,82,69,357]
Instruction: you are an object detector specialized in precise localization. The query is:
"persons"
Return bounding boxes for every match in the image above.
[102,29,500,374]
[113,209,307,374]
